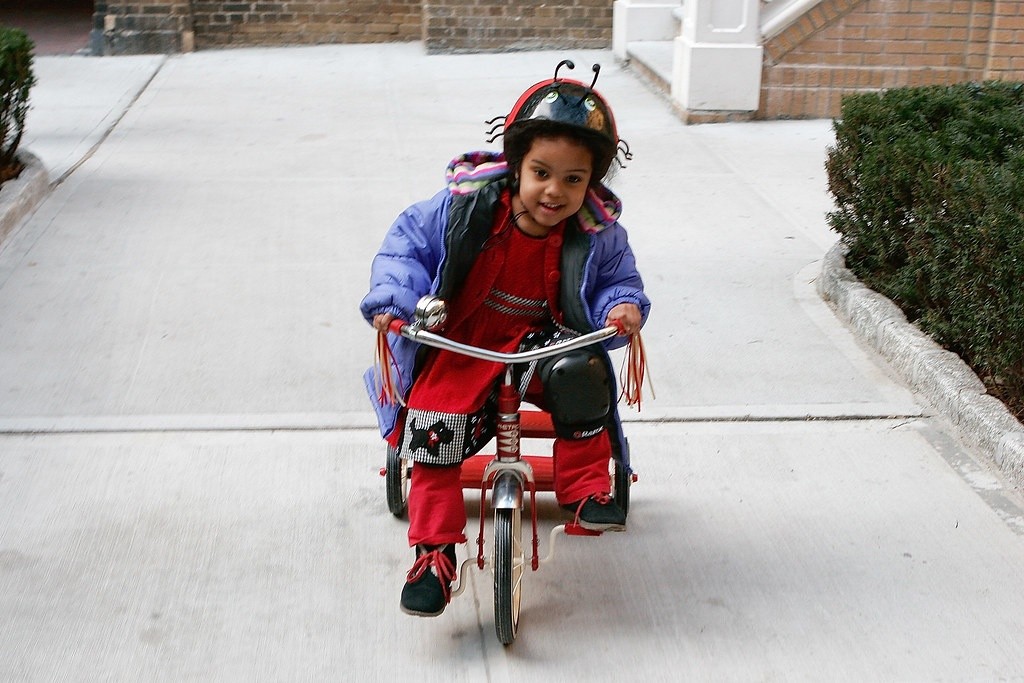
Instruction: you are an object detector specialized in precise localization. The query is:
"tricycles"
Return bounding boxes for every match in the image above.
[374,301,640,651]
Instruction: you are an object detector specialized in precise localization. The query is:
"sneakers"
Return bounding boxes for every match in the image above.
[558,490,627,534]
[400,539,458,618]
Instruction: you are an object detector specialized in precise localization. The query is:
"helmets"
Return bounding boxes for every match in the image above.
[503,78,618,189]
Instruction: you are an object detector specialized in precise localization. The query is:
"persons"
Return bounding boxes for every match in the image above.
[343,56,652,616]
